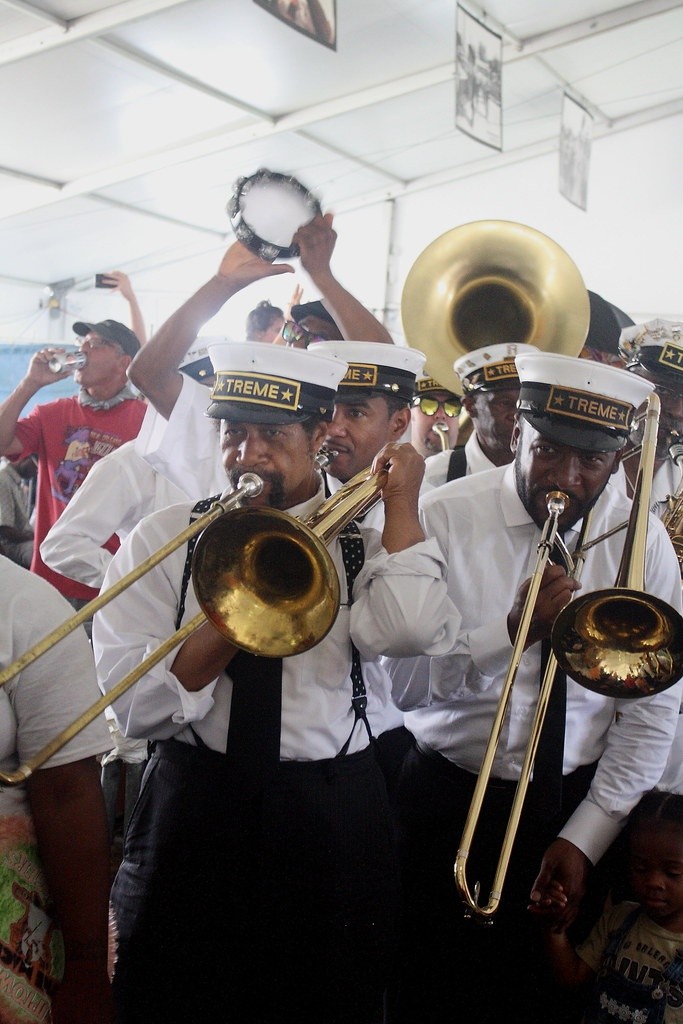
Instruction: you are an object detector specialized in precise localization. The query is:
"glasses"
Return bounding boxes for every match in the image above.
[282,321,328,348]
[75,336,115,351]
[410,395,461,417]
[654,382,683,401]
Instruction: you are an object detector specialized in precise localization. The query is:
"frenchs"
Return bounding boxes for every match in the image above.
[399,218,591,452]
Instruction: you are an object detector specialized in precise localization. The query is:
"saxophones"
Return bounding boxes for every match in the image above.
[313,446,340,471]
[658,427,683,578]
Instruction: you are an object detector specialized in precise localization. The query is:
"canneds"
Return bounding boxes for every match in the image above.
[48,350,87,374]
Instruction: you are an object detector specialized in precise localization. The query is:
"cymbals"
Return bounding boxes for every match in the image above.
[227,166,324,265]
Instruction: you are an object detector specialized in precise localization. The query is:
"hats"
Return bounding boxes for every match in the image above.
[616,317,683,385]
[72,319,140,358]
[413,370,451,392]
[206,341,347,426]
[453,342,538,396]
[513,353,655,451]
[288,299,335,323]
[306,341,428,401]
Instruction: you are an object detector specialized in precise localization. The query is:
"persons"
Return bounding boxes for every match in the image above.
[0,209,683,1024]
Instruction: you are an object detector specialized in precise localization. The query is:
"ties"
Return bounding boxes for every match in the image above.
[213,647,283,855]
[529,536,568,830]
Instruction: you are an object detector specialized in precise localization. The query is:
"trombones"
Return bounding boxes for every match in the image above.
[451,392,682,929]
[2,463,392,790]
